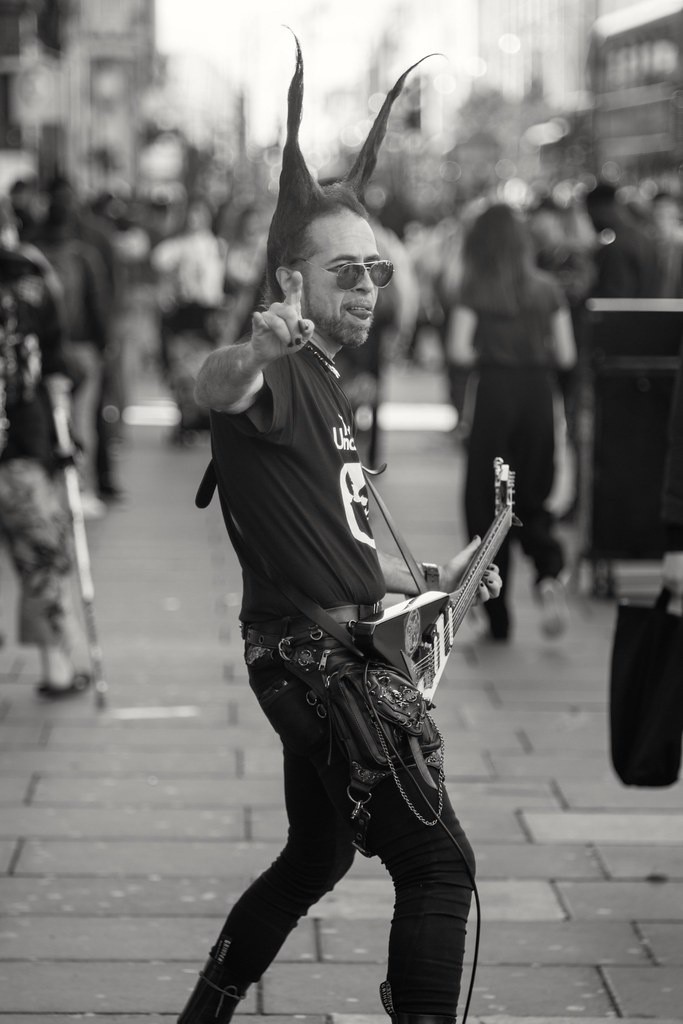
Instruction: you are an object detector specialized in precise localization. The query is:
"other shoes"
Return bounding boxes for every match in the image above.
[36,673,88,699]
[541,581,566,637]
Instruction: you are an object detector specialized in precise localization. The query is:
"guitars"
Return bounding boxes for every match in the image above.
[354,455,516,705]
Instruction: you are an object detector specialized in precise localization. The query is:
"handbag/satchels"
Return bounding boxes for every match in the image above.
[312,630,446,823]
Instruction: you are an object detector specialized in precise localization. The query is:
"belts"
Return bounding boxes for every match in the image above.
[246,600,381,639]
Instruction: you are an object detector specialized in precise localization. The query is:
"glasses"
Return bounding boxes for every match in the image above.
[288,256,394,288]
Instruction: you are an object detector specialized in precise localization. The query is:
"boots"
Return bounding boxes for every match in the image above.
[177,956,254,1023]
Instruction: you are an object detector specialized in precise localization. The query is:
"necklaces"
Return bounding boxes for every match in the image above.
[313,337,335,365]
[308,345,341,378]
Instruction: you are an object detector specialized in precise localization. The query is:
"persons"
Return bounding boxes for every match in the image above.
[381,180,683,528]
[447,201,575,646]
[0,177,261,501]
[0,201,91,701]
[176,190,502,1024]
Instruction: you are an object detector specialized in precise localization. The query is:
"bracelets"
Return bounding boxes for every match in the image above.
[422,563,440,592]
